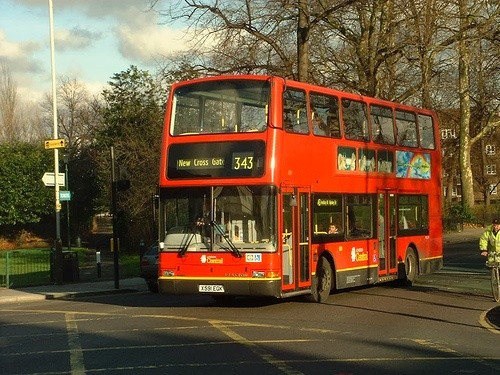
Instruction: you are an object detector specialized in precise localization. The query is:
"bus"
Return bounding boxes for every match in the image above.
[153,74,446,304]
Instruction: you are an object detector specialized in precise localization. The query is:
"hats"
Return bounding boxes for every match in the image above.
[492,219,500,224]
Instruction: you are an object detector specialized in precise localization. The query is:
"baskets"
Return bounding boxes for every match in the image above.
[485,261,500,268]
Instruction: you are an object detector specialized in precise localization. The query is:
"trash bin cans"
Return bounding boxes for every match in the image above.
[49,251,80,283]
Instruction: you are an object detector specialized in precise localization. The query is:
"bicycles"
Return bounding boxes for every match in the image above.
[483,251,500,303]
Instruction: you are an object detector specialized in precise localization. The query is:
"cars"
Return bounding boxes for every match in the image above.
[139,243,159,293]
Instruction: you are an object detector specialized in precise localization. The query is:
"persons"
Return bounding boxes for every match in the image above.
[192,199,221,238]
[479,219,500,284]
[327,224,338,234]
